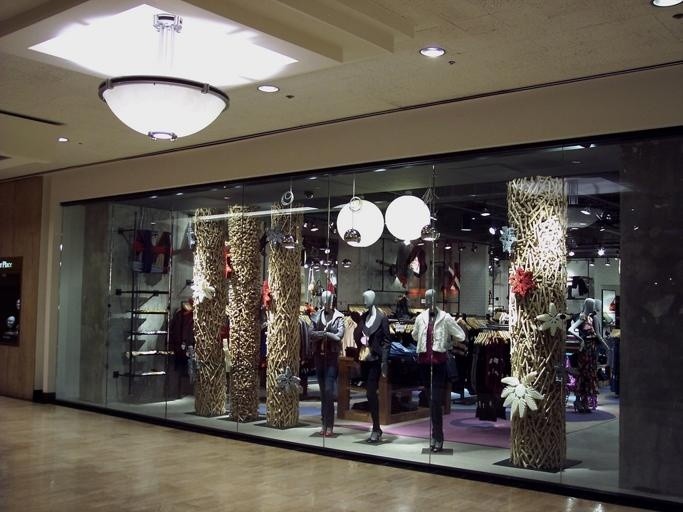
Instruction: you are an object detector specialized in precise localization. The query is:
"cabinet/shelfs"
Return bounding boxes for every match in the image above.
[112,287,170,379]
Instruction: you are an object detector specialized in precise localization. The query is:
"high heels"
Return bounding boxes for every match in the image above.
[366,429,382,443]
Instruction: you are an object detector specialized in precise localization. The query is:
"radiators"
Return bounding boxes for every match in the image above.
[457,316,481,331]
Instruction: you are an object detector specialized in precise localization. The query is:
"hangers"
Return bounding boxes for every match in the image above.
[473,329,511,347]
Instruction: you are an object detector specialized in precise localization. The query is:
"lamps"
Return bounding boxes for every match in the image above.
[97,12,230,142]
[307,219,319,232]
[334,170,432,248]
[460,212,473,232]
[589,257,595,267]
[480,200,492,217]
[604,257,610,267]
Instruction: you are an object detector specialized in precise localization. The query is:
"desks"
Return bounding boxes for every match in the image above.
[337,355,452,425]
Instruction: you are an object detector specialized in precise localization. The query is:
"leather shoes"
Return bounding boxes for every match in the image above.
[430,440,443,452]
[319,425,333,436]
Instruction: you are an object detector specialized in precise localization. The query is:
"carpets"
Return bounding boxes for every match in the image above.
[296,408,513,449]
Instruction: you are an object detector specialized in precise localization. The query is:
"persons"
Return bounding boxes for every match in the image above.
[307,289,345,436]
[568,297,609,414]
[411,289,466,452]
[353,290,391,443]
